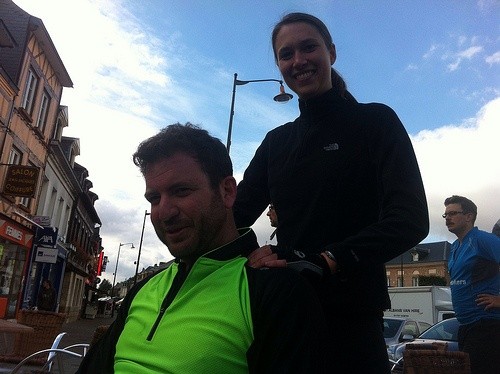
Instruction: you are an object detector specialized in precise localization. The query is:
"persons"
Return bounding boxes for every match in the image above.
[74,13,429,374]
[444,195,500,373]
[40,280,57,312]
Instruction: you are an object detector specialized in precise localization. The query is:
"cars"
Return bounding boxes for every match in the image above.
[383,318,459,366]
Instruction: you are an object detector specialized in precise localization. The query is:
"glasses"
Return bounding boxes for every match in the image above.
[269,206,275,212]
[442,211,465,218]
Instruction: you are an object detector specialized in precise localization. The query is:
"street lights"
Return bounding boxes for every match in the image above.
[110,243,135,299]
[227,73,294,156]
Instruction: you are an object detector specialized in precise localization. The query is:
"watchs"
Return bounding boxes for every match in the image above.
[325,251,336,261]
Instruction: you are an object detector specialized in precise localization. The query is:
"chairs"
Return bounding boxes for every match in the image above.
[0,310,110,373]
[403,344,470,374]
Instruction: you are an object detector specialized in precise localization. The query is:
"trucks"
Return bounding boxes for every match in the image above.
[383,286,456,326]
